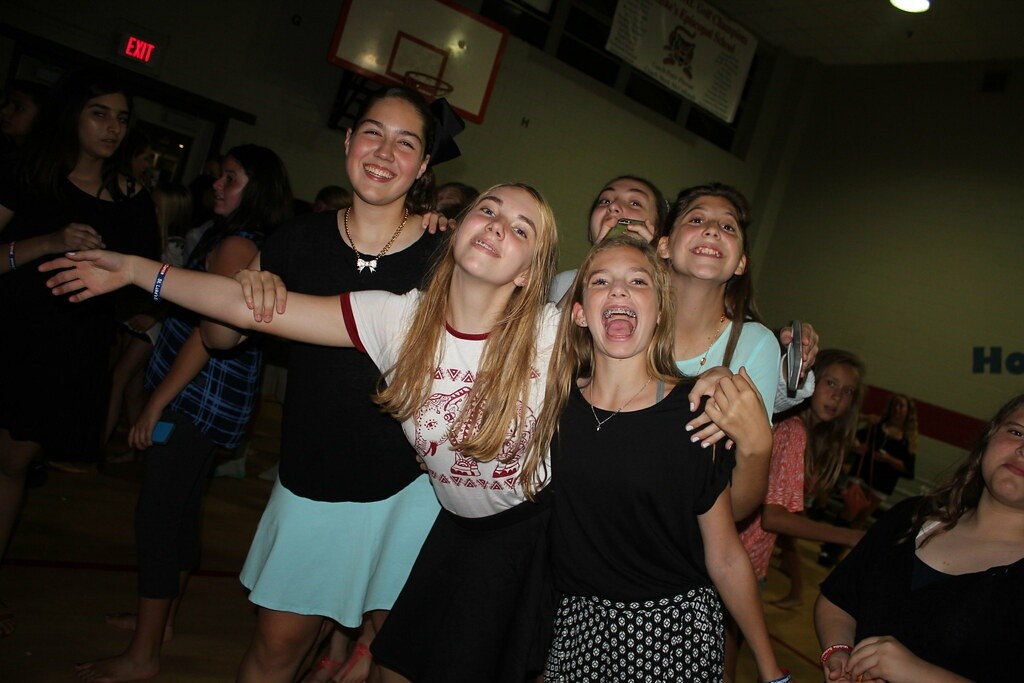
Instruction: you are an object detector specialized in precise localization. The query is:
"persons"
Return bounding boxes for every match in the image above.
[40,182,566,683]
[817,393,920,567]
[74,141,301,683]
[200,80,486,683]
[543,174,821,415]
[313,186,352,212]
[0,86,48,231]
[812,389,1024,682]
[654,184,783,521]
[421,180,480,234]
[118,129,164,183]
[0,81,166,548]
[705,347,866,683]
[515,234,796,683]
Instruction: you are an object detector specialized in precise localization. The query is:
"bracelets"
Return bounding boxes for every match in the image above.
[757,666,793,683]
[151,262,173,303]
[820,644,855,664]
[8,240,16,273]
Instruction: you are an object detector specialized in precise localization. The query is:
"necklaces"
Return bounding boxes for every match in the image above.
[589,367,655,432]
[344,204,409,275]
[692,312,726,379]
